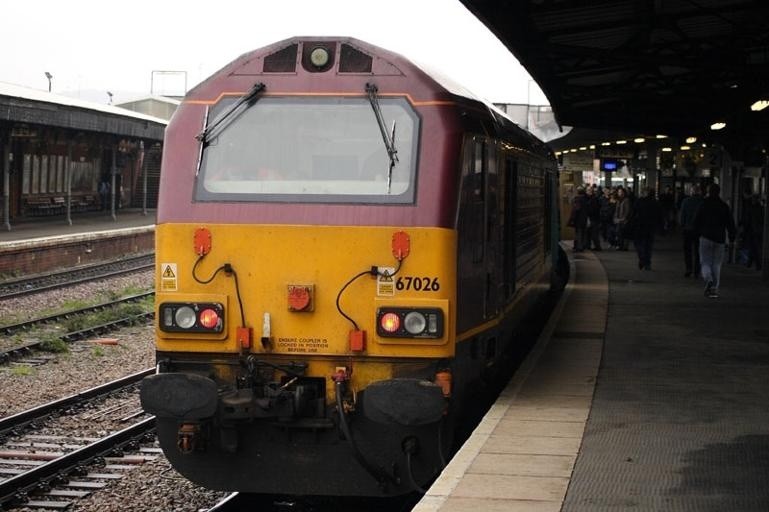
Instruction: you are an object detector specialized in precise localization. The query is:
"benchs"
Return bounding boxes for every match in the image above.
[25,195,101,214]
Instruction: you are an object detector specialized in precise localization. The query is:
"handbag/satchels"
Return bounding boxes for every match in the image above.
[622,219,638,240]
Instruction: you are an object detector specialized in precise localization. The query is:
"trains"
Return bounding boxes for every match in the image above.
[135,35,568,500]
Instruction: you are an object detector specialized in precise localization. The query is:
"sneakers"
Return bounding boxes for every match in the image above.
[707,287,721,299]
[703,276,714,295]
[571,242,629,252]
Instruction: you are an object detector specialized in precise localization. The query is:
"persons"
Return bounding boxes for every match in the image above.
[566,183,765,298]
[99,177,110,211]
